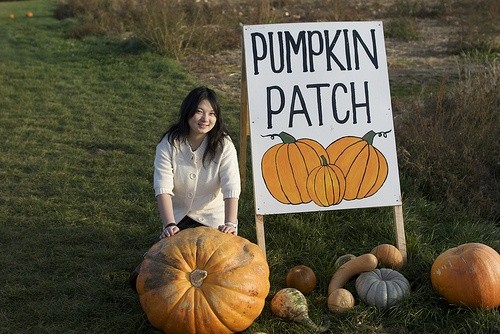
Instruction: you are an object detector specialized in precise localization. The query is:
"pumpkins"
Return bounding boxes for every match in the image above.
[271,235,500,330]
[255,124,394,202]
[135,225,271,333]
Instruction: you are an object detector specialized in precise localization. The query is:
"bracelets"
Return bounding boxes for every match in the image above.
[226,222,236,228]
[164,223,177,229]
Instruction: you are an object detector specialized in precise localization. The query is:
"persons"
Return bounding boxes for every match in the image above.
[152,86,242,238]
